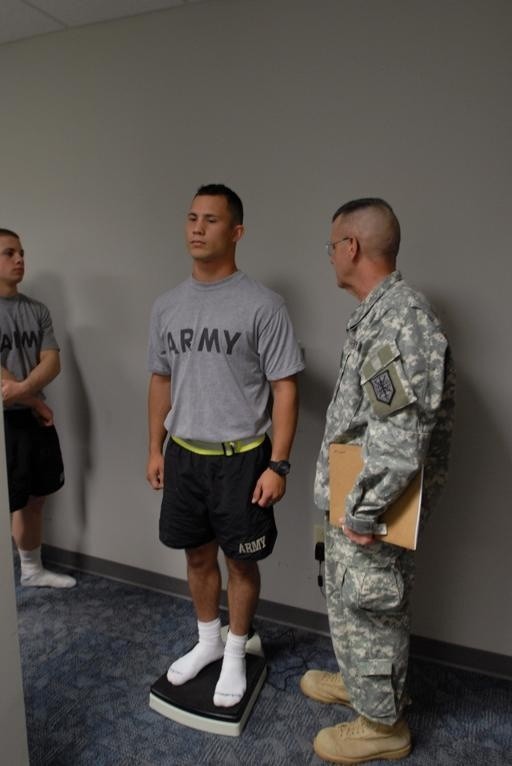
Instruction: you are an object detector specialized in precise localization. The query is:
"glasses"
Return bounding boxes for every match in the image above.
[325,237,352,255]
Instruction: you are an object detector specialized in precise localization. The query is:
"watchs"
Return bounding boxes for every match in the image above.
[267,459,291,478]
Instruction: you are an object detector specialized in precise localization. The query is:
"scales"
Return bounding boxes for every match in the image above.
[149,638,269,738]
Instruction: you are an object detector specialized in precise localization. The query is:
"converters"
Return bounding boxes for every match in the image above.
[314,542,325,562]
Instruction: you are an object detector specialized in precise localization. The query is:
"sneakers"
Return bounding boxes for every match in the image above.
[312,716,413,764]
[298,669,399,706]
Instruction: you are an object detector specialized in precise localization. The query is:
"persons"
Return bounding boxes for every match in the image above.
[145,183,306,708]
[300,197,457,766]
[0,228,78,589]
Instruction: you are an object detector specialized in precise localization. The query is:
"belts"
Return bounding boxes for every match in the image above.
[173,437,263,456]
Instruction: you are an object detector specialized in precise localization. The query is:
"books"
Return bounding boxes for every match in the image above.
[328,442,424,551]
